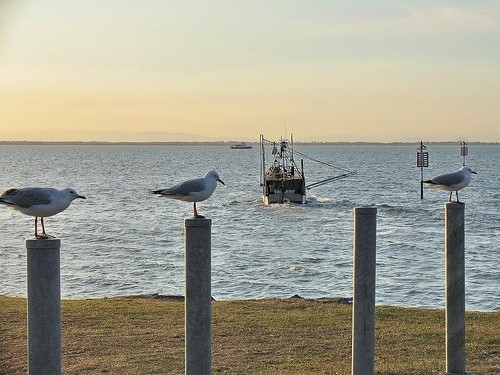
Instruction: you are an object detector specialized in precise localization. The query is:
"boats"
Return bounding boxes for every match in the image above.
[260,132,357,205]
[230,141,253,149]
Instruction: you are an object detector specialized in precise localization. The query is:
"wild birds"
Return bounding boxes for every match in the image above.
[423,166,477,203]
[151,171,226,218]
[0,186,87,239]
